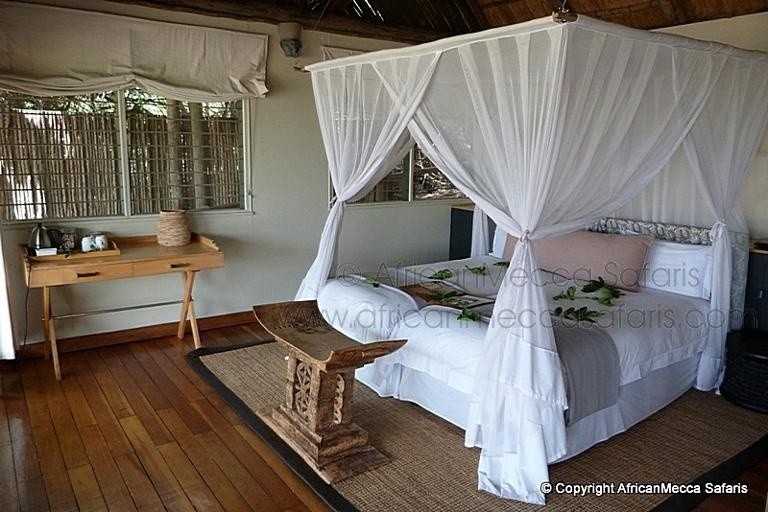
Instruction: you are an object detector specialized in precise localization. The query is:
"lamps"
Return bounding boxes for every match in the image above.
[276,19,303,57]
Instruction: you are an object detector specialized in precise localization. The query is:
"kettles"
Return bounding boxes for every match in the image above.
[25,222,62,254]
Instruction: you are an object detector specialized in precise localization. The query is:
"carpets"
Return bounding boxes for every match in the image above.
[184,334,768,512]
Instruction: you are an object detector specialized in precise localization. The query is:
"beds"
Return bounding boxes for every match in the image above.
[292,4,767,465]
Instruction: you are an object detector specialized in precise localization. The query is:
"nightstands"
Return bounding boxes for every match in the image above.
[449,203,497,260]
[719,239,765,413]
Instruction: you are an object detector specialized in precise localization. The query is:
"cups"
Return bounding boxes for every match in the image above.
[80,235,95,251]
[96,233,108,250]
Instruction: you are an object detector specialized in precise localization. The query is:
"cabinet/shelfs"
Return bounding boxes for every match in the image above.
[18,231,225,381]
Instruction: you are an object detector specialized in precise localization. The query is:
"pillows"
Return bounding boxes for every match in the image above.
[621,228,714,301]
[499,226,654,294]
